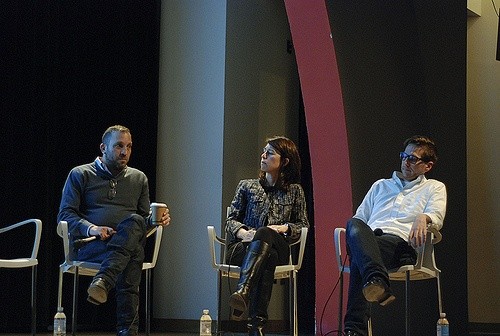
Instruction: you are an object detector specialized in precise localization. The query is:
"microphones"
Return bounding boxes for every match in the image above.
[374,228,383,236]
[74,234,98,247]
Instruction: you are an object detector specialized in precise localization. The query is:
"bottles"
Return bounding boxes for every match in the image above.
[53,307,66,336]
[437,313,449,336]
[199,309,212,336]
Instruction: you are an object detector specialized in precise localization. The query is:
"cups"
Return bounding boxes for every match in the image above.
[149,202,167,224]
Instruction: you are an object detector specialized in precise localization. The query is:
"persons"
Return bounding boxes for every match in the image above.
[343,135,447,336]
[57,125,171,336]
[224,136,311,336]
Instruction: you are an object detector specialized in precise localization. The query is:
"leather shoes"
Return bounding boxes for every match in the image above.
[117,327,139,336]
[87,279,112,305]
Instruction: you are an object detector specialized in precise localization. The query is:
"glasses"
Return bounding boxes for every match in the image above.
[400,152,421,164]
[107,179,118,200]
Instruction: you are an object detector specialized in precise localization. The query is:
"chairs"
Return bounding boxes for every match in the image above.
[0,219,42,336]
[57,221,163,336]
[208,207,308,336]
[334,224,442,336]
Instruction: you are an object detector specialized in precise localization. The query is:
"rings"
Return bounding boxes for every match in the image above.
[277,229,279,232]
[169,218,171,221]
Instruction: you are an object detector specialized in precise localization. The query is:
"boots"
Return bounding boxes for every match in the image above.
[229,240,270,321]
[246,270,277,336]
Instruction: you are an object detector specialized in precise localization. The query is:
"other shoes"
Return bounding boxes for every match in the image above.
[344,328,360,336]
[362,276,398,306]
